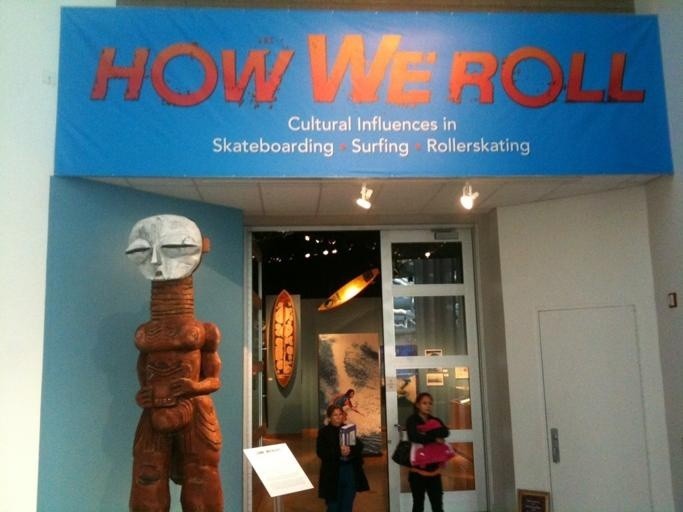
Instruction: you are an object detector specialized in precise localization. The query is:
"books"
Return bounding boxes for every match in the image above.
[339,423,357,460]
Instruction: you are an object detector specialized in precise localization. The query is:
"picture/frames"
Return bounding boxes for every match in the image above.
[517,489,550,512]
[425,349,443,386]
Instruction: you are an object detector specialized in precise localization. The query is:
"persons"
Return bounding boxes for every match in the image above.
[315,404,371,511]
[331,388,366,420]
[405,392,450,511]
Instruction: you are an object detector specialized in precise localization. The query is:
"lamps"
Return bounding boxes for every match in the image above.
[356,184,373,210]
[461,182,480,210]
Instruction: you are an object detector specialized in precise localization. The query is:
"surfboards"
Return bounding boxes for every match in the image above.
[273,290,297,388]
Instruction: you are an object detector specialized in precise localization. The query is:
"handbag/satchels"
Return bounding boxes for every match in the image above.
[391,439,424,467]
